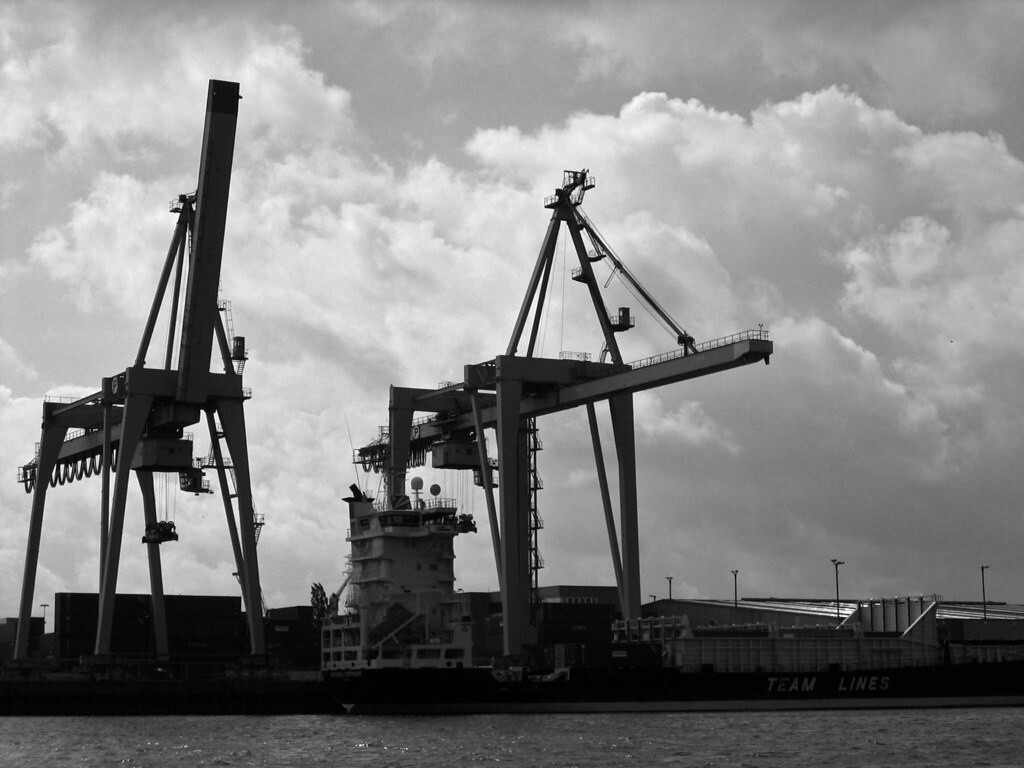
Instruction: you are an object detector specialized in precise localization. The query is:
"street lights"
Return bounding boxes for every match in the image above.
[731,570,738,608]
[831,558,844,618]
[981,565,990,619]
[666,576,673,600]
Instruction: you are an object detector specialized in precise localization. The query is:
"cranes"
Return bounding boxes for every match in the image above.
[14,82,268,661]
[355,167,773,664]
